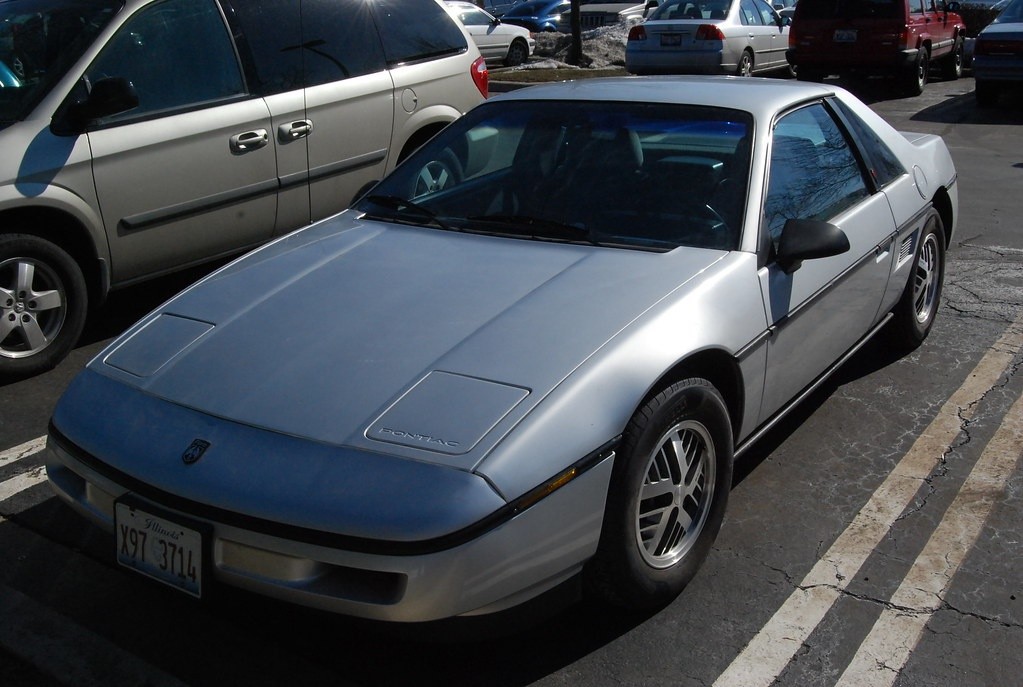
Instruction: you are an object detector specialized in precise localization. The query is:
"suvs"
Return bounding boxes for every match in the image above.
[786,0,968,96]
[557,0,699,37]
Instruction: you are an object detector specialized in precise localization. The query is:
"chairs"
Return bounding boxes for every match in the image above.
[729,135,820,244]
[545,125,643,232]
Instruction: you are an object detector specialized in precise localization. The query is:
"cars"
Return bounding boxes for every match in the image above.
[623,0,798,78]
[750,0,799,28]
[972,0,1023,101]
[482,0,522,21]
[499,0,572,36]
[442,0,537,67]
[44,73,960,625]
[0,0,499,380]
